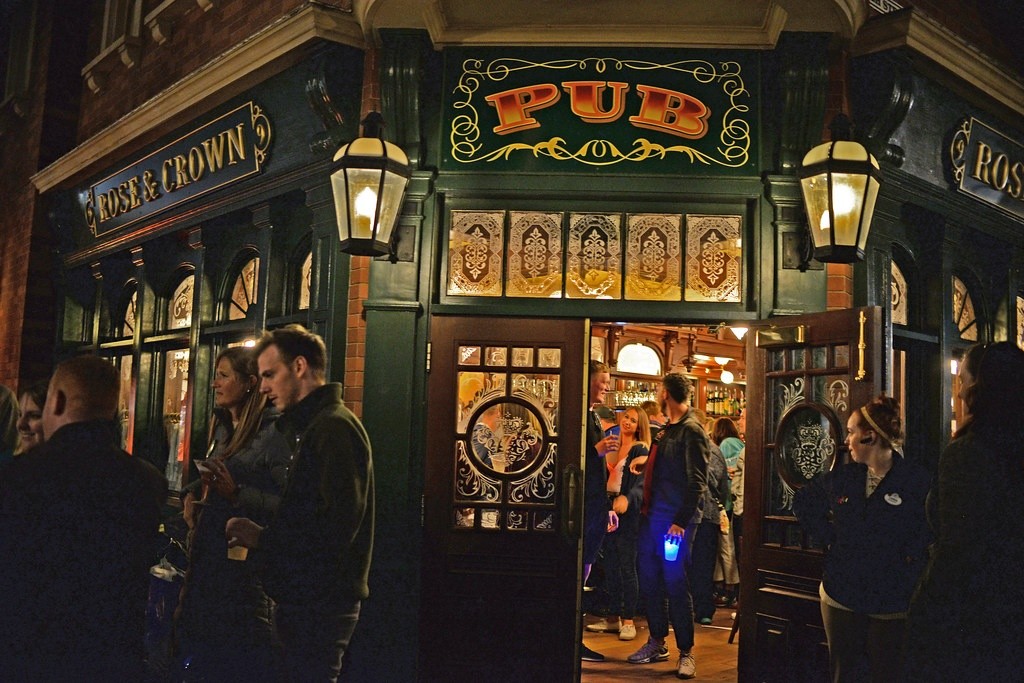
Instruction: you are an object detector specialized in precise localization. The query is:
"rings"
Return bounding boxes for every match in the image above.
[211,475,217,481]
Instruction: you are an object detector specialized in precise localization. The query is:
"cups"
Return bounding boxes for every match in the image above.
[725,457,738,471]
[608,430,622,451]
[664,534,681,561]
[227,545,248,560]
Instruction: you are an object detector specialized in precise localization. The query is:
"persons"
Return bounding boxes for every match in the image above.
[658,388,746,623]
[896,341,1024,683]
[0,354,170,683]
[794,399,931,683]
[150,323,375,683]
[629,372,711,678]
[458,358,668,661]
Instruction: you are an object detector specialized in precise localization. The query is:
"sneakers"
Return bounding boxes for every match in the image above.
[676,649,696,678]
[627,639,669,664]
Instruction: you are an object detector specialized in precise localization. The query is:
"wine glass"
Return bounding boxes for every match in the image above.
[190,459,221,506]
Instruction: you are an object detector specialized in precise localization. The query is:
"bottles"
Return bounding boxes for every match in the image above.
[615,379,658,406]
[705,385,746,416]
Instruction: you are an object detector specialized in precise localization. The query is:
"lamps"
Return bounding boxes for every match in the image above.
[329,111,416,264]
[783,111,884,272]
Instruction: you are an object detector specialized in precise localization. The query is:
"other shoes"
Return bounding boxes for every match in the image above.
[713,595,738,606]
[701,617,711,624]
[619,619,637,640]
[581,645,605,661]
[586,618,619,632]
